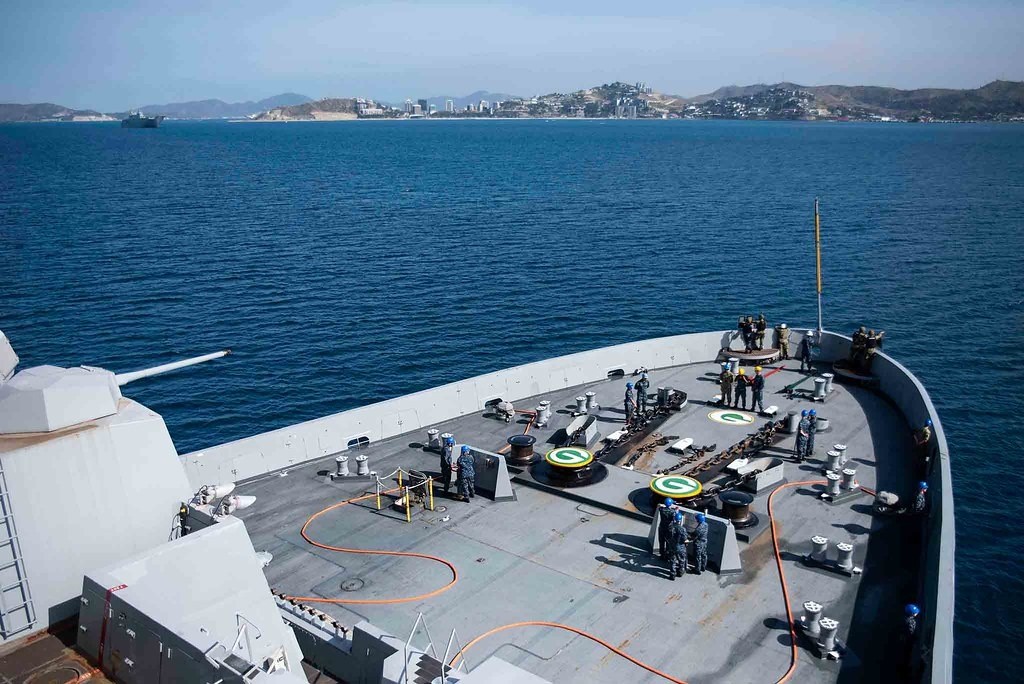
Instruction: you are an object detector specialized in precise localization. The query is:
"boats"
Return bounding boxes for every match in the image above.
[122,109,167,129]
[0,326,960,684]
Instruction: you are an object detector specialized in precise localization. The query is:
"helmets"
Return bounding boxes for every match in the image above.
[739,369,745,375]
[461,446,470,452]
[626,383,633,387]
[755,366,762,371]
[809,409,816,416]
[924,418,933,426]
[724,365,730,369]
[806,331,813,336]
[905,604,920,614]
[675,513,682,520]
[917,482,929,490]
[780,323,786,329]
[694,513,705,522]
[801,410,808,416]
[664,497,673,506]
[446,438,454,443]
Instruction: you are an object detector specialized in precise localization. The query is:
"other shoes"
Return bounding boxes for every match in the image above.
[468,493,475,498]
[678,573,683,577]
[463,498,471,503]
[669,575,675,580]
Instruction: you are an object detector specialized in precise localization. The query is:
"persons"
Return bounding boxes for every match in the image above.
[658,498,679,560]
[775,323,791,360]
[741,317,753,354]
[625,382,638,425]
[901,604,922,660]
[719,364,733,408]
[863,330,885,374]
[911,480,929,515]
[849,327,868,371]
[667,513,688,580]
[753,314,767,350]
[733,368,749,411]
[912,418,932,471]
[457,446,476,503]
[794,409,810,464]
[635,373,651,416]
[750,366,765,414]
[691,514,708,576]
[805,409,817,456]
[440,437,454,496]
[800,330,814,373]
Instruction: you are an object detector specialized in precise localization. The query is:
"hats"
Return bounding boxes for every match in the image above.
[642,373,649,379]
